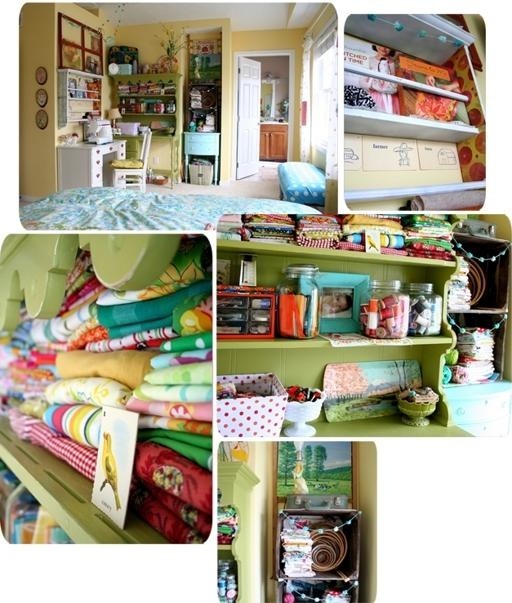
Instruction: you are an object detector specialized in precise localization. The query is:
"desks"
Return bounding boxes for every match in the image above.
[183,132,221,185]
[57,140,126,188]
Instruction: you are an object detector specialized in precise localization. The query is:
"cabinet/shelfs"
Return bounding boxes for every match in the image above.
[109,73,183,189]
[218,463,260,603]
[260,124,288,161]
[186,83,218,132]
[275,506,362,603]
[217,240,474,437]
[344,14,486,202]
[57,68,103,129]
[443,232,512,388]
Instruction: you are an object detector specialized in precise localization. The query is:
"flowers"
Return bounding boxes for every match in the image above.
[153,23,190,57]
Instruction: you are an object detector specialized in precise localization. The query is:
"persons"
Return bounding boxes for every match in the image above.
[374,55,396,115]
[424,74,459,91]
[358,42,400,117]
[319,292,352,316]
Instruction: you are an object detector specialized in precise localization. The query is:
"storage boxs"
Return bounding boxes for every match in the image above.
[217,372,289,437]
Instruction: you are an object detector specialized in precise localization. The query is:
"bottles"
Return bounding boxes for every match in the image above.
[275,263,322,340]
[361,281,442,339]
[217,562,236,603]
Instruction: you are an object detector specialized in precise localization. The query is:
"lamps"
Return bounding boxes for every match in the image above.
[108,108,122,136]
[266,72,273,83]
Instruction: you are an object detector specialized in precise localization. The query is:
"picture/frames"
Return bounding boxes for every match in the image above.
[267,441,360,603]
[299,271,371,333]
[58,13,103,76]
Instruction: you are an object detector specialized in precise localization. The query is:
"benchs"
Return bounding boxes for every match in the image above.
[277,162,326,205]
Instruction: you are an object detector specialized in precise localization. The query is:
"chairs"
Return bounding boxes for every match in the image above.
[113,129,152,189]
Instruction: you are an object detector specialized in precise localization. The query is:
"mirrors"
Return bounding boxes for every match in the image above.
[261,80,280,117]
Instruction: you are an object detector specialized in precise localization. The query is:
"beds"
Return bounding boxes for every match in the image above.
[19,188,324,230]
